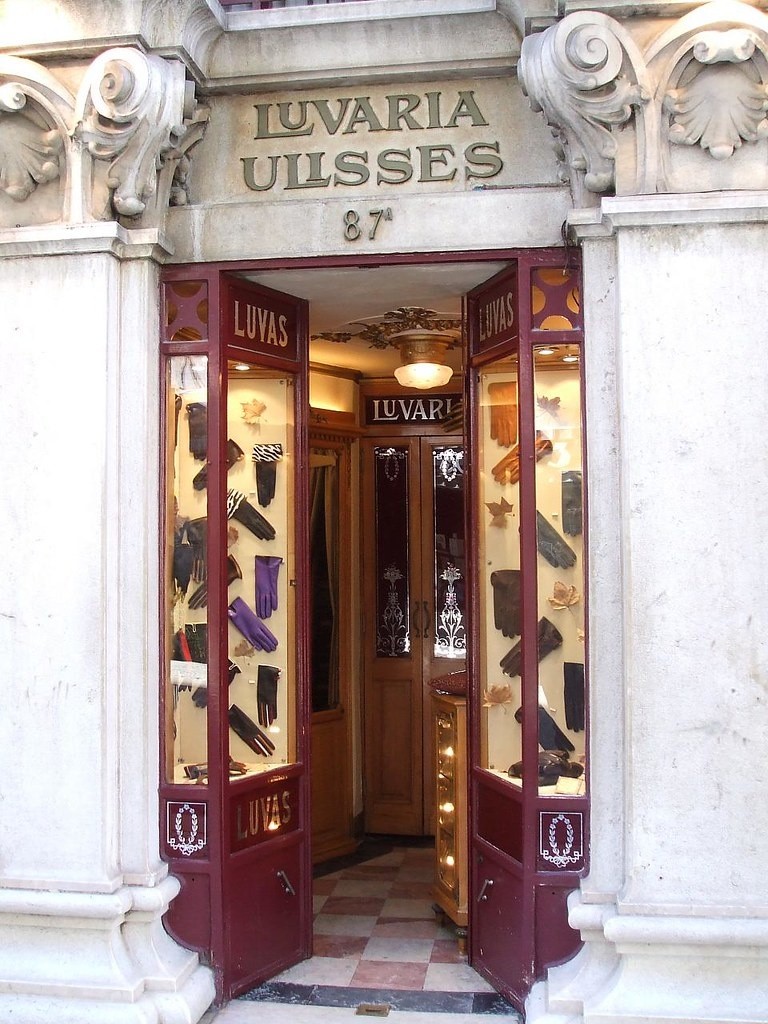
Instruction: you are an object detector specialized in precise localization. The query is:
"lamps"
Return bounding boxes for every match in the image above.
[358,306,467,390]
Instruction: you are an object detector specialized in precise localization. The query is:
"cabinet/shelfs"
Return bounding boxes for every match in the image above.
[428,690,466,955]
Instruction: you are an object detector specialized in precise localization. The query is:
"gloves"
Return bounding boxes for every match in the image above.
[490,570,521,639]
[491,430,553,485]
[186,403,207,461]
[251,443,283,508]
[518,510,577,569]
[564,662,584,732]
[172,516,242,709]
[228,703,275,757]
[508,752,584,787]
[499,616,562,678]
[193,439,245,491]
[257,664,282,728]
[255,555,283,619]
[227,488,276,541]
[561,471,582,536]
[488,381,517,448]
[183,756,247,780]
[228,596,278,653]
[514,704,575,752]
